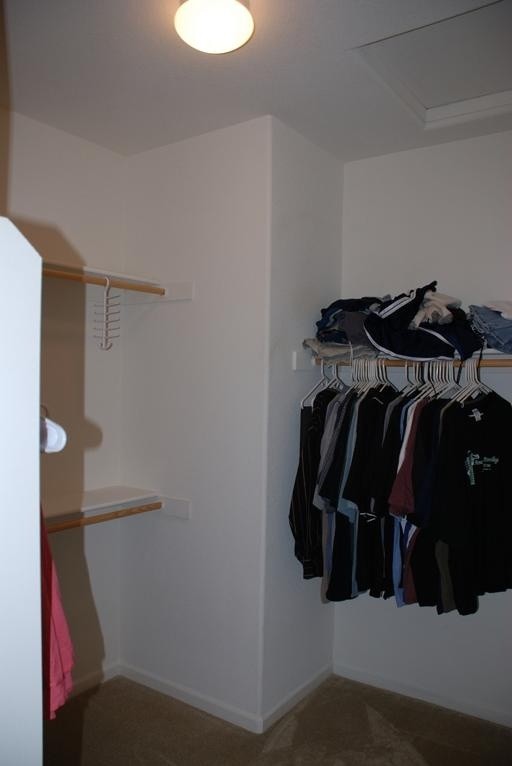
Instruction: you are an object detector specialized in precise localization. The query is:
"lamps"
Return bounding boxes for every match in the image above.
[173,0,254,55]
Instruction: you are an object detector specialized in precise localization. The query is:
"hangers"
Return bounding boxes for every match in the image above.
[300,355,494,412]
